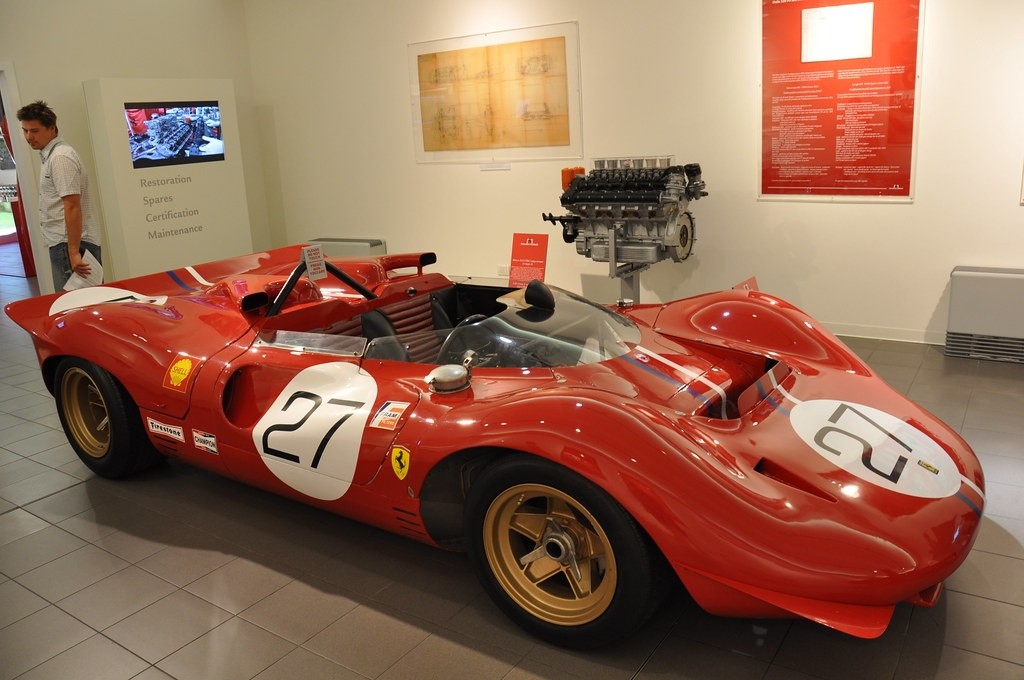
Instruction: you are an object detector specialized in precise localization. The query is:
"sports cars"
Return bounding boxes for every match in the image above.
[5,243,989,646]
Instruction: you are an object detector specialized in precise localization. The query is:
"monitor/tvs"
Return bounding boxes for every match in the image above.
[124,100,225,169]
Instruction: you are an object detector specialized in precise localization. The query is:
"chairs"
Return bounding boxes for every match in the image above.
[365,292,460,363]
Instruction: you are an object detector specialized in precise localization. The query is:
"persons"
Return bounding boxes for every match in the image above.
[17,103,105,295]
